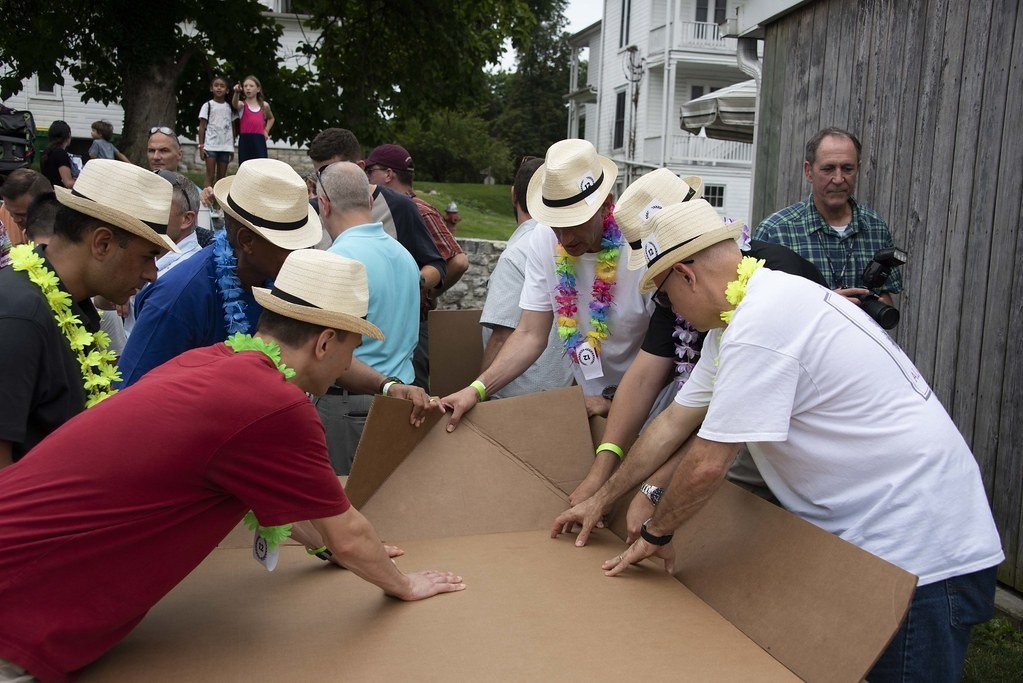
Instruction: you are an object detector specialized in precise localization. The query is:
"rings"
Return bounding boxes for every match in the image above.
[430,399,434,402]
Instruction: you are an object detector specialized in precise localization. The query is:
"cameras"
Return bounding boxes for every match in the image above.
[842,246,908,330]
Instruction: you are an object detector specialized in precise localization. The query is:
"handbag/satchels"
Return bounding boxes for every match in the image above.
[0,107,37,173]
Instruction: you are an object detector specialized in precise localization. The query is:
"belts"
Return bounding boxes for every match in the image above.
[326,384,361,396]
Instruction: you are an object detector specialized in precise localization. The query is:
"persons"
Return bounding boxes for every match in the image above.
[89,121,223,359]
[0,249,467,683]
[315,162,423,477]
[550,199,1008,683]
[112,159,438,427]
[752,127,902,305]
[40,120,77,189]
[199,76,274,188]
[0,159,178,469]
[568,169,829,544]
[308,128,469,393]
[438,138,652,432]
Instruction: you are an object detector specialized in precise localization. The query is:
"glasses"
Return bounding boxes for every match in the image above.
[651,259,694,308]
[364,167,399,176]
[316,164,331,203]
[153,168,191,211]
[148,127,181,147]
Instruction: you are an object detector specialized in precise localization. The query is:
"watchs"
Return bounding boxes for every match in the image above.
[640,483,663,504]
[380,376,405,392]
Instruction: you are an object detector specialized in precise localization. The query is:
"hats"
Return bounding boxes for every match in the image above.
[526,139,618,228]
[213,158,323,249]
[251,249,385,341]
[613,168,704,270]
[53,159,183,255]
[363,144,415,172]
[638,199,743,295]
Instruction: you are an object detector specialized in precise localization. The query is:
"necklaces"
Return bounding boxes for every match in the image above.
[223,333,296,542]
[555,204,622,364]
[8,242,122,409]
[672,217,753,389]
[214,228,250,333]
[712,258,763,386]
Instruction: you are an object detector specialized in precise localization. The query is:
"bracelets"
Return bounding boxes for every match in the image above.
[198,144,204,147]
[317,550,331,560]
[596,442,624,460]
[305,546,326,554]
[470,381,486,402]
[640,517,674,546]
[383,381,396,396]
